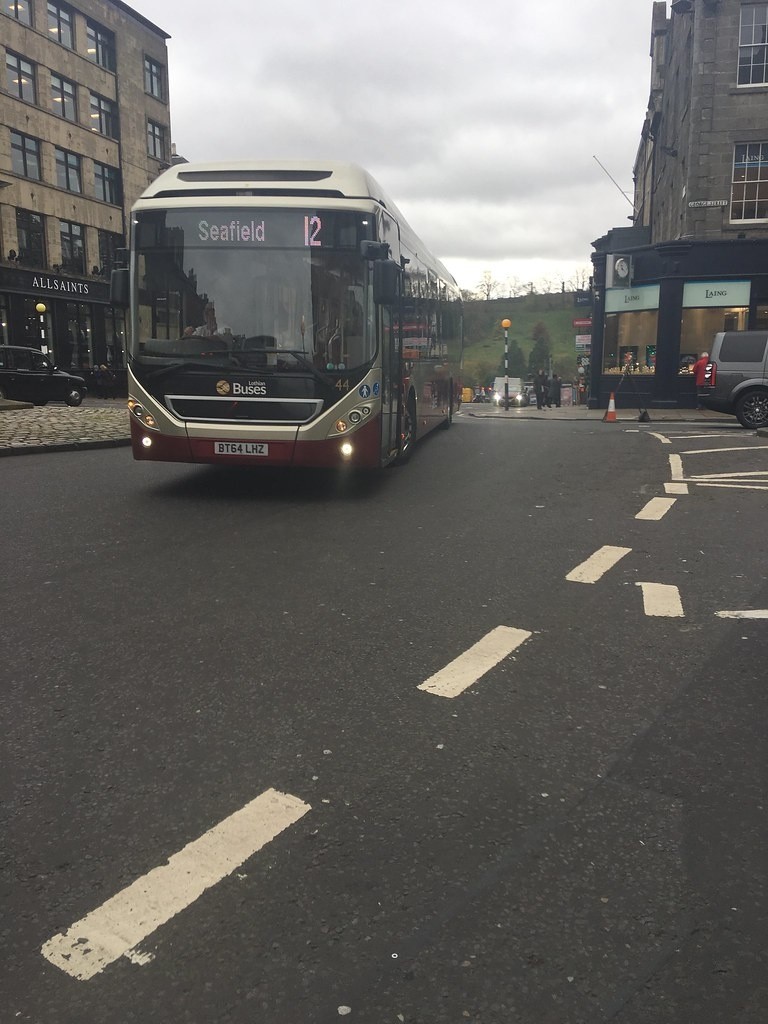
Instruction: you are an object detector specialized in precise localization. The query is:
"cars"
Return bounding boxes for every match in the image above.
[0,345,87,406]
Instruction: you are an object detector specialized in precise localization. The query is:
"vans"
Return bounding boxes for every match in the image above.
[493,377,528,407]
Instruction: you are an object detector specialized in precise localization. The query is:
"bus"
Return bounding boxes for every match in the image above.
[107,160,465,472]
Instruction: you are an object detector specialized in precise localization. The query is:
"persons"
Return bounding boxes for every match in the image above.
[94,364,117,399]
[479,387,485,404]
[180,301,236,338]
[693,352,710,411]
[533,368,563,410]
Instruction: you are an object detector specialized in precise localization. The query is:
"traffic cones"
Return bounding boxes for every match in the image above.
[603,392,621,423]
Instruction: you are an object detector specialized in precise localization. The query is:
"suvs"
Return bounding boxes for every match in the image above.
[697,330,768,430]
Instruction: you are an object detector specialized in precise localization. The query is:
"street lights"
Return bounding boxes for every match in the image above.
[501,318,511,410]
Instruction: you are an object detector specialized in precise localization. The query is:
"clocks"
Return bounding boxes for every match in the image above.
[616,258,630,278]
